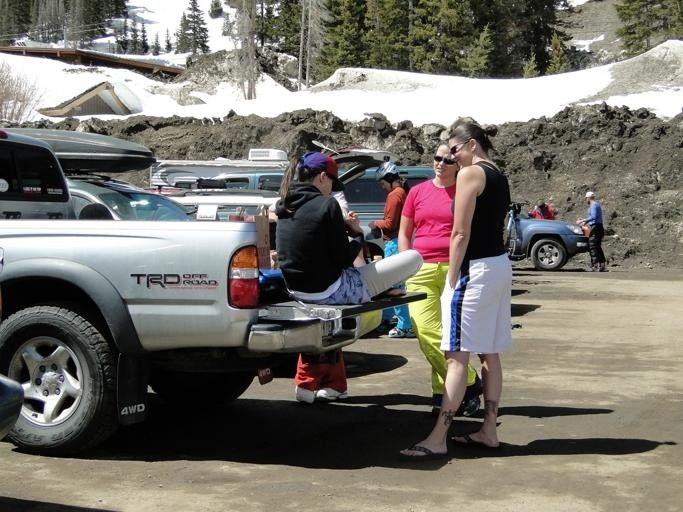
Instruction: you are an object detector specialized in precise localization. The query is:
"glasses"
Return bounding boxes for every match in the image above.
[449,141,468,154]
[433,155,457,165]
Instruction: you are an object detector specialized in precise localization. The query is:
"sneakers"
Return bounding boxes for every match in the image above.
[461,372,483,417]
[389,326,415,338]
[431,394,441,418]
[316,387,348,401]
[294,384,315,405]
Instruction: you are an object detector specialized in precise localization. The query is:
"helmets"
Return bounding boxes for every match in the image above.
[375,161,399,181]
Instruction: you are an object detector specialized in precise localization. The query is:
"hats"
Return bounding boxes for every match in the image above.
[585,192,595,198]
[298,151,346,192]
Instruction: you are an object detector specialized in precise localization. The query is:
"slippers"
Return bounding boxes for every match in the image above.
[450,434,502,451]
[398,446,450,461]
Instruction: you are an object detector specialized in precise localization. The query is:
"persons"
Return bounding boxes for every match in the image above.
[360,161,417,333]
[578,190,608,273]
[399,141,484,416]
[275,151,422,304]
[528,196,557,220]
[281,128,348,403]
[400,115,507,462]
